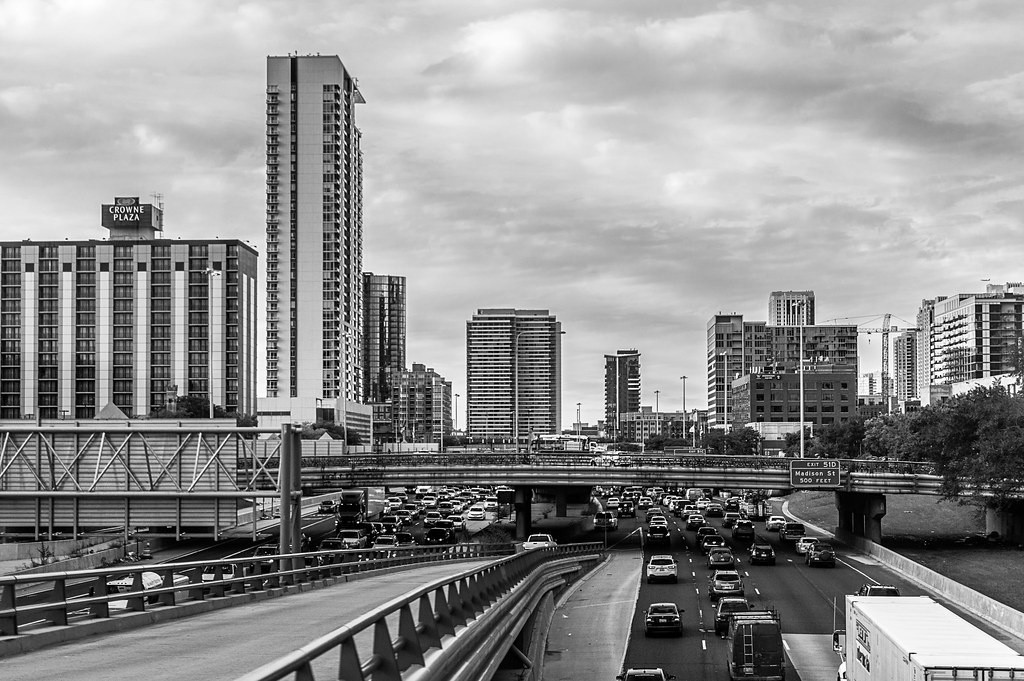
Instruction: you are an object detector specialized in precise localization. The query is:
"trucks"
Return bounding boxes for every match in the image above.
[337,487,385,523]
[832,584,1024,681]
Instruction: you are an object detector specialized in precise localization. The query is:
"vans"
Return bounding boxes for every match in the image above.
[721,607,783,681]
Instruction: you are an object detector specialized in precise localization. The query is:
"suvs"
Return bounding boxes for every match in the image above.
[805,542,836,567]
[747,542,776,566]
[707,569,745,602]
[423,511,443,528]
[433,520,455,540]
[315,516,416,557]
[711,596,755,636]
[645,555,679,583]
[250,532,311,574]
[202,564,247,584]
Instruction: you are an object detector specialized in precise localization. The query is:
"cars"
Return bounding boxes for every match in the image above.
[384,485,510,518]
[616,668,676,681]
[525,533,557,550]
[445,514,466,531]
[706,546,735,570]
[592,486,821,555]
[642,602,686,637]
[467,505,486,521]
[318,500,337,514]
[482,496,502,510]
[424,528,450,546]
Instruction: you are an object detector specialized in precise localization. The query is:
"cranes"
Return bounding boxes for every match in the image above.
[817,313,919,405]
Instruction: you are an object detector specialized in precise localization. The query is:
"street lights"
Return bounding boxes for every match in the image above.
[515,330,566,462]
[342,333,347,447]
[654,391,661,435]
[792,300,804,458]
[576,403,580,442]
[719,351,728,452]
[455,394,460,438]
[680,376,687,439]
[511,411,532,458]
[440,383,448,453]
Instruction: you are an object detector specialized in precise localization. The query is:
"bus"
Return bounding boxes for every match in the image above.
[514,434,605,451]
[88,571,190,610]
